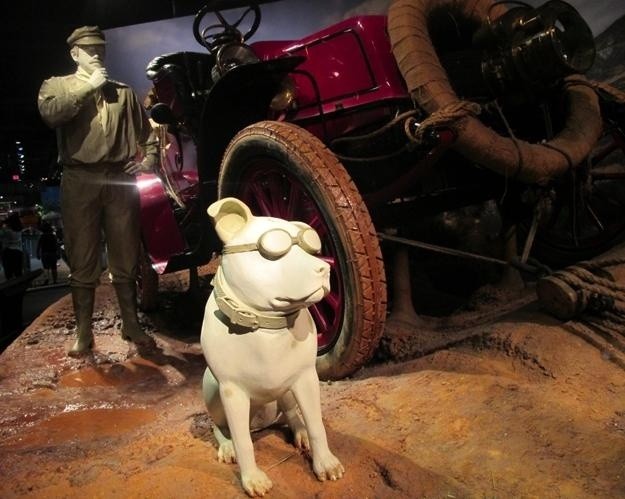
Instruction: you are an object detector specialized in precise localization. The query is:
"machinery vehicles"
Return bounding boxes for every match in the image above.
[132,0,624,380]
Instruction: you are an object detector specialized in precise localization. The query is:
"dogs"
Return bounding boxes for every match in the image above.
[200,197,345,497]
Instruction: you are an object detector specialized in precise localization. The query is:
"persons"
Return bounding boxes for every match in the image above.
[35,221,62,285]
[1,208,30,302]
[32,23,164,363]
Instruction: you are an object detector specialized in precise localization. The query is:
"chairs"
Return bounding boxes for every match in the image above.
[144,51,214,101]
